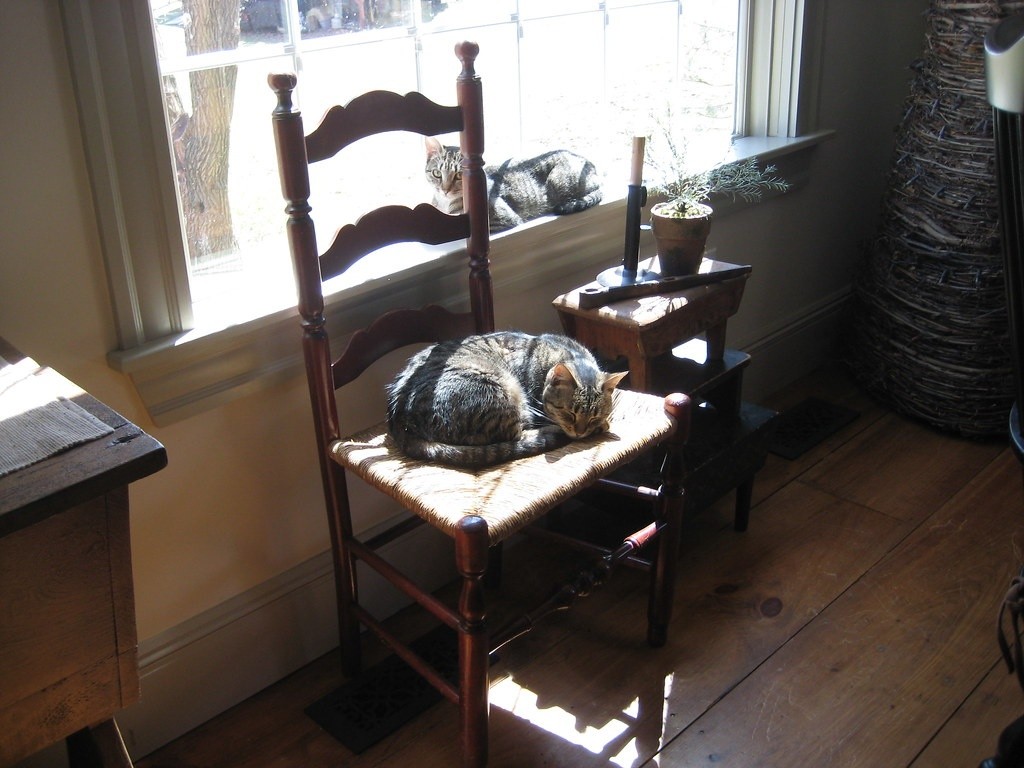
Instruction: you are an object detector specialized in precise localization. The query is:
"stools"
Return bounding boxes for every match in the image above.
[571,396,782,557]
[593,337,751,460]
[552,253,752,394]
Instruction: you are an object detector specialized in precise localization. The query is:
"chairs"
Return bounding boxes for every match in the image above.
[266,41,691,768]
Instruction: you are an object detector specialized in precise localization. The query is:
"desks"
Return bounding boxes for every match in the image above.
[0,335,171,768]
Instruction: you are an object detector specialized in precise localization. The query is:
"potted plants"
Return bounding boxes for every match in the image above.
[643,112,792,276]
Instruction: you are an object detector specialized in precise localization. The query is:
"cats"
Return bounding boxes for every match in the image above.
[425,135,604,231]
[384,331,629,468]
